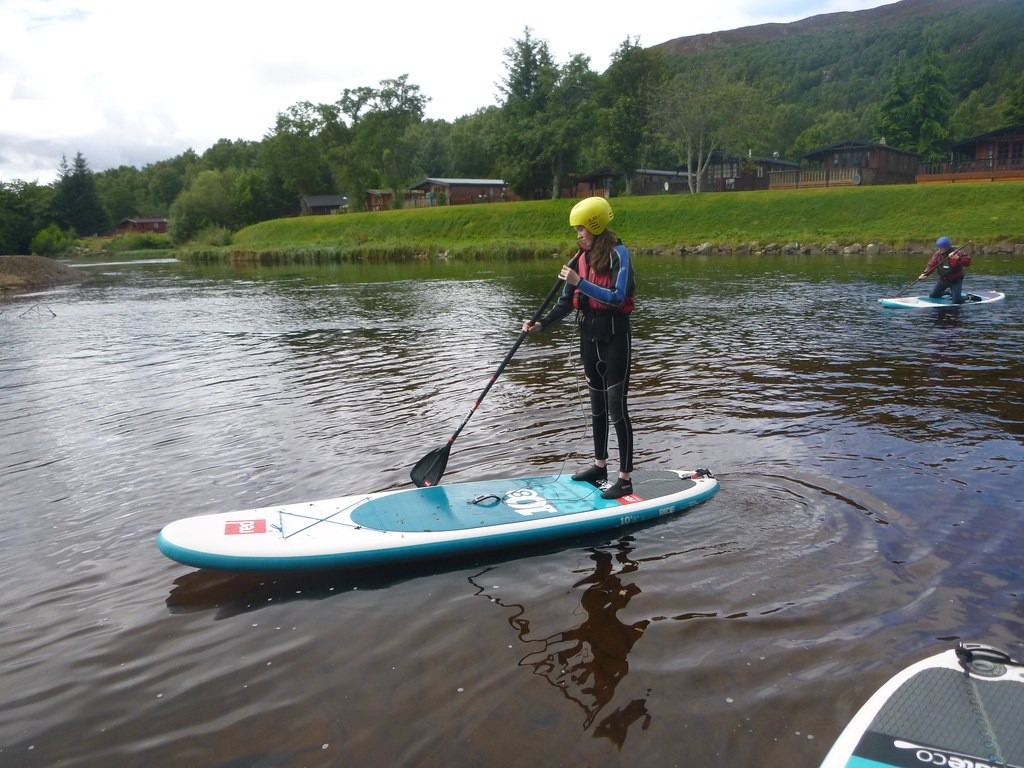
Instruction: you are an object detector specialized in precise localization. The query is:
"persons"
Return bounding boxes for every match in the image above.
[522,196,635,498]
[918,236,982,303]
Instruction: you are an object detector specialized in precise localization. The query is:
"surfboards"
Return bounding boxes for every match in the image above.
[816,639,1022,768]
[156,465,721,574]
[880,290,1005,308]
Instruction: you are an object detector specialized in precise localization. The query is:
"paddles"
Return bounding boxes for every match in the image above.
[409,242,587,488]
[893,240,973,298]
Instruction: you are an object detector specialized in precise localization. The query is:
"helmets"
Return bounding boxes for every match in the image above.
[569,196,615,236]
[936,236,951,248]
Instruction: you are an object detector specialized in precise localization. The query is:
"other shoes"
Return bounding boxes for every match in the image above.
[571,464,608,481]
[942,291,951,295]
[600,477,633,498]
[966,294,982,301]
